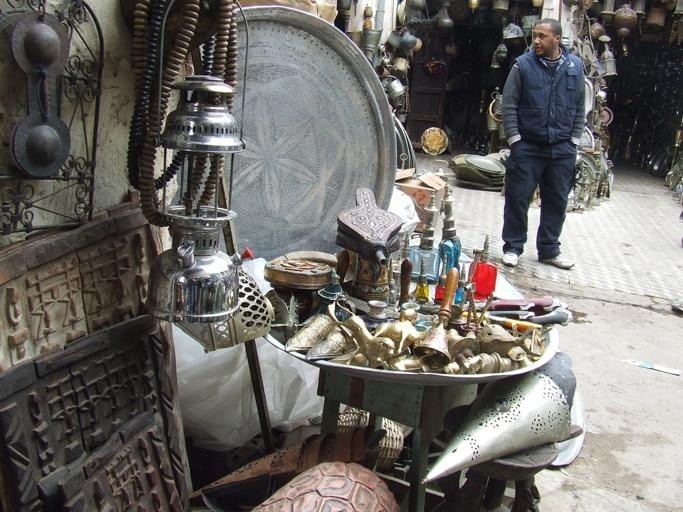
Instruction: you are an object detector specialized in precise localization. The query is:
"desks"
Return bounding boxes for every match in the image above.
[259,255,562,511]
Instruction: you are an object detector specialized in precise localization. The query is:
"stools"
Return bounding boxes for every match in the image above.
[439,401,560,511]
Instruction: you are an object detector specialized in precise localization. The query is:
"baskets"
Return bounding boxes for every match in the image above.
[337,404,405,472]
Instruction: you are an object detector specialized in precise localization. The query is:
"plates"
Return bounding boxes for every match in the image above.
[420,127,448,155]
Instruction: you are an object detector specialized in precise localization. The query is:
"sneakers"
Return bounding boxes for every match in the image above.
[539,255,574,270]
[502,251,519,267]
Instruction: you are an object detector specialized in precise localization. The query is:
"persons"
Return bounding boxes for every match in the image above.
[501,18,586,269]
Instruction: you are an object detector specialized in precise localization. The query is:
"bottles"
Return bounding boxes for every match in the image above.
[409,229,497,305]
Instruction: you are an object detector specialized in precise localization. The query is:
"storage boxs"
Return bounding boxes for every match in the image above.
[395,167,447,233]
